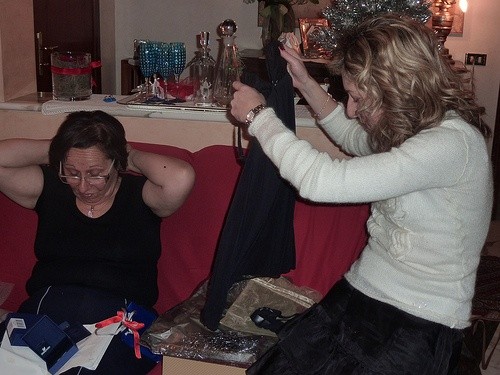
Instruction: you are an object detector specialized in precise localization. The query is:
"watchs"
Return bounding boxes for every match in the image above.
[245,104,267,126]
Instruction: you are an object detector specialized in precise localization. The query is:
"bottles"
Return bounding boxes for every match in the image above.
[213,18,242,108]
[190,31,216,107]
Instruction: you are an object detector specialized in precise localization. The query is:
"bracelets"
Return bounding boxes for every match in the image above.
[314,96,330,116]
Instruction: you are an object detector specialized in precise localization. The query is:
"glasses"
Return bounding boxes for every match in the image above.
[58,157,117,187]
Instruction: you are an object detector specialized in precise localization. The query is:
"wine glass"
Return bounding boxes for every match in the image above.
[140,42,187,104]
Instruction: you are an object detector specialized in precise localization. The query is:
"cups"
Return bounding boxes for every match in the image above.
[50,50,92,102]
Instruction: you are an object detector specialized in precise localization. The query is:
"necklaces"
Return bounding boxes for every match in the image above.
[78,200,110,218]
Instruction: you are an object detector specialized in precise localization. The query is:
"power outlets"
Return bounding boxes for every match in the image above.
[464,53,487,66]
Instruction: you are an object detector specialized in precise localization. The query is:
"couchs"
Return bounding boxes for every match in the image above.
[0,140,370,375]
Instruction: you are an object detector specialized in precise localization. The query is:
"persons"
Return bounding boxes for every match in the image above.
[229,14,494,375]
[0,109,195,375]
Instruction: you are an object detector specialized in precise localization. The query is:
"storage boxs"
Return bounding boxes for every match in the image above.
[6,311,41,345]
[22,313,79,375]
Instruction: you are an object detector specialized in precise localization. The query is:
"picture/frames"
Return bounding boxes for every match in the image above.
[298,17,333,59]
